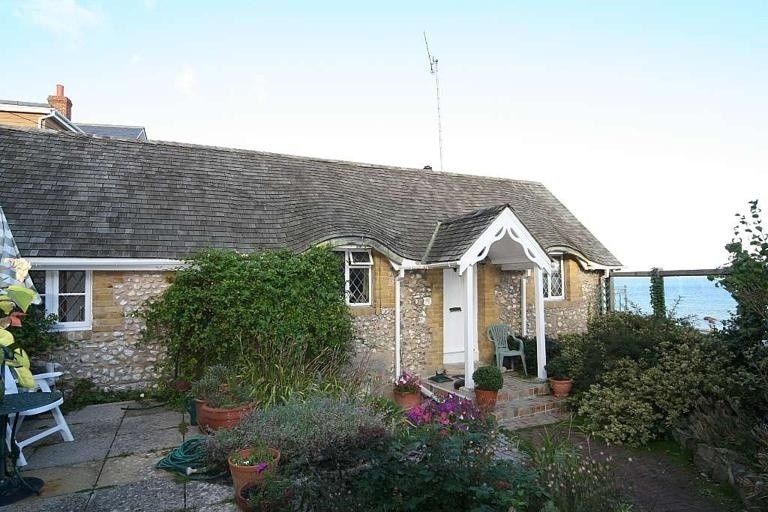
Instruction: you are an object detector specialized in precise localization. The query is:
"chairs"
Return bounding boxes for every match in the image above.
[0,362,74,467]
[487,324,529,377]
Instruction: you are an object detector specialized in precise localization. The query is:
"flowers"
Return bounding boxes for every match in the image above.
[407,393,485,439]
[232,444,275,475]
[389,371,422,394]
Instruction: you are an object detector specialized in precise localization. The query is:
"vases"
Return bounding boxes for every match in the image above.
[393,391,423,410]
[228,448,280,492]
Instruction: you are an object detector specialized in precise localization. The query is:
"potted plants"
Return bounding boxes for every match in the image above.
[235,477,292,511]
[133,243,359,435]
[315,424,391,479]
[544,355,573,398]
[472,366,503,408]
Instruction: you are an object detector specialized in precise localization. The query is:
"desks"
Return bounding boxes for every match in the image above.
[0,391,61,507]
